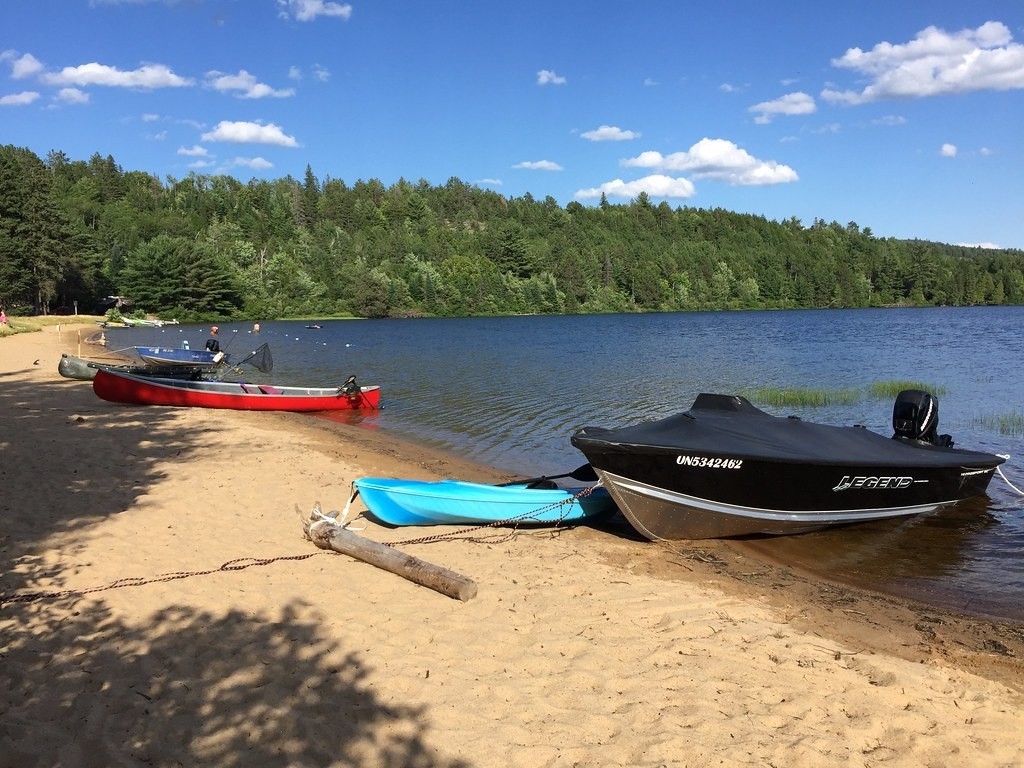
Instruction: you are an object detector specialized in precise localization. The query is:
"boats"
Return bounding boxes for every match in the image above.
[350,472,614,530]
[132,346,232,371]
[58,354,227,382]
[95,313,180,329]
[89,360,381,414]
[568,391,1009,549]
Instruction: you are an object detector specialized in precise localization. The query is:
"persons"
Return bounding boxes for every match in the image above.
[0,309,6,324]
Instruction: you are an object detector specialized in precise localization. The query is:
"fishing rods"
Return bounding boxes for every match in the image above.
[223,314,250,352]
